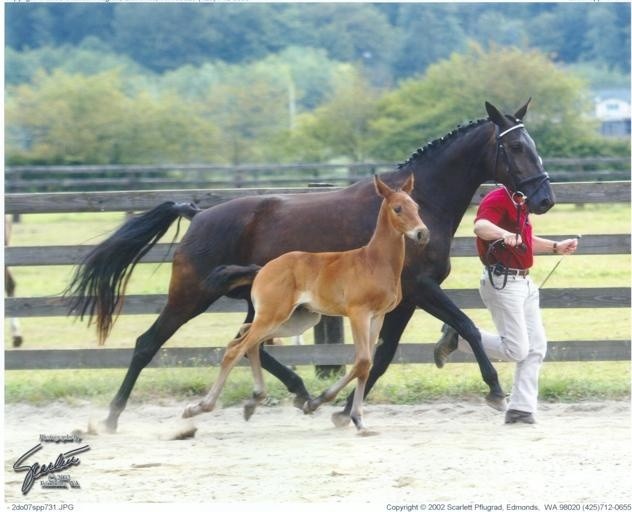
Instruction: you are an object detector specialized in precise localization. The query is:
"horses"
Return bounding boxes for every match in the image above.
[55,94,557,433]
[180,171,431,437]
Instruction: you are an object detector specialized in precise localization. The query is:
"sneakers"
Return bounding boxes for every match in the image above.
[434,324,458,368]
[504,409,535,423]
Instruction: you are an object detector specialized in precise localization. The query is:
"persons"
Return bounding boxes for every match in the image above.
[434,185,579,425]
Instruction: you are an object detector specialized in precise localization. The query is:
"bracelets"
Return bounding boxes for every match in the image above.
[553,241,557,254]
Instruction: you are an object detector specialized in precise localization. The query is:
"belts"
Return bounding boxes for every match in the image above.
[484,265,530,276]
